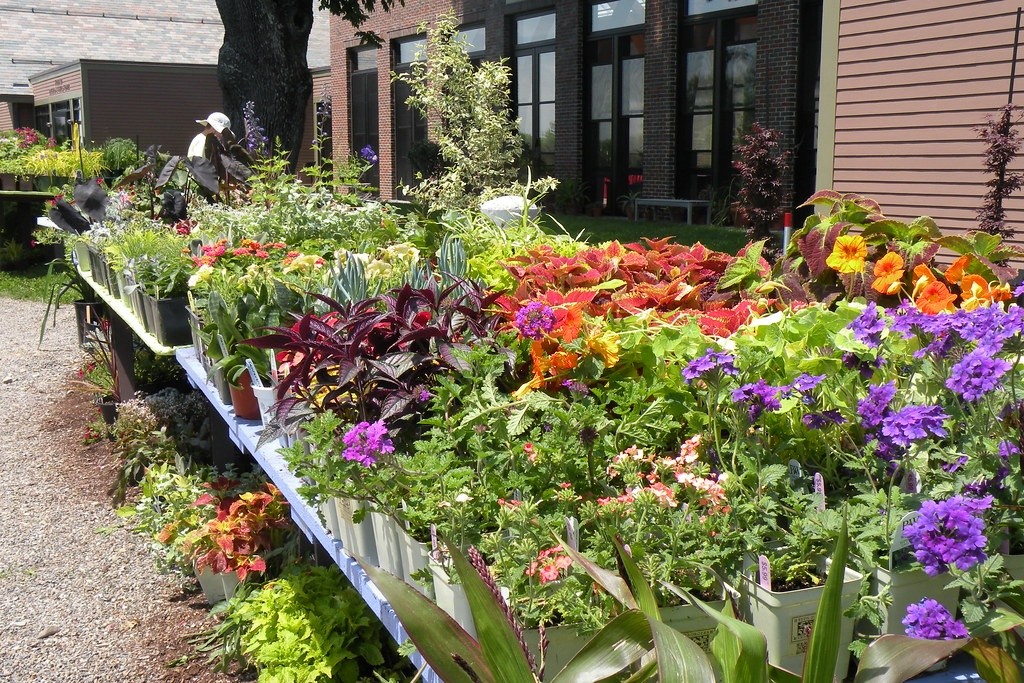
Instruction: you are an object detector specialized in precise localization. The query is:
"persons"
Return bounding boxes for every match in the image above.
[187,111,236,161]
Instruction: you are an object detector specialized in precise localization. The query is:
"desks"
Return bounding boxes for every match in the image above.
[77,266,190,404]
[176,347,445,683]
[0,191,55,195]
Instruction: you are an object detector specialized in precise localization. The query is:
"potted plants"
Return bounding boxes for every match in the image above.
[552,180,591,217]
[136,230,193,347]
[37,258,104,349]
[616,189,643,220]
[713,177,751,227]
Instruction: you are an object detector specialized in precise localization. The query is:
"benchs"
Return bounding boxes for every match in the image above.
[634,198,712,226]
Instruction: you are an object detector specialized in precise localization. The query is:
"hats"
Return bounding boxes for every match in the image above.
[195,112,236,142]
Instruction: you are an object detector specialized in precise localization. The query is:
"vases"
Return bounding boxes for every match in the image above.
[845,548,959,671]
[429,551,509,643]
[37,176,52,192]
[521,624,631,683]
[396,521,435,604]
[229,371,262,420]
[1000,552,1024,640]
[192,559,227,608]
[736,546,864,680]
[369,499,403,582]
[96,393,120,443]
[250,382,292,430]
[659,582,741,683]
[53,176,68,190]
[0,173,17,191]
[18,174,35,191]
[221,570,240,602]
[331,476,379,567]
[83,317,102,344]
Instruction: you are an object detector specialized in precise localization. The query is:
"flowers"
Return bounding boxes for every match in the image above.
[0,81,1024,683]
